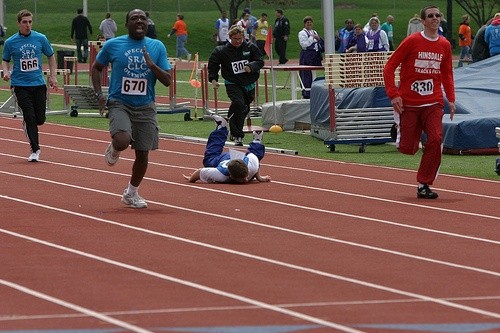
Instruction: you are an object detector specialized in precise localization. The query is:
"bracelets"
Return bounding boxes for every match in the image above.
[95,92,102,99]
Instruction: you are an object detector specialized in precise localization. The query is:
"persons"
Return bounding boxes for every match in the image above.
[255,13,268,56]
[92,8,173,208]
[272,10,290,64]
[208,25,265,146]
[0,24,4,46]
[183,115,271,183]
[233,9,258,43]
[145,12,156,39]
[383,6,456,198]
[335,13,395,53]
[215,11,231,46]
[71,8,92,63]
[298,16,324,99]
[408,13,447,37]
[99,13,117,41]
[458,13,500,67]
[168,14,192,61]
[2,9,58,162]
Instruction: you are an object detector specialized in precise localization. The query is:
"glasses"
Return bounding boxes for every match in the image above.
[424,13,442,18]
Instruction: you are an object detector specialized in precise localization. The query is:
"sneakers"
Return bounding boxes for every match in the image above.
[211,114,228,128]
[390,122,399,140]
[28,149,41,162]
[234,137,243,146]
[229,130,236,141]
[416,186,438,199]
[252,129,264,140]
[121,188,148,208]
[104,141,120,166]
[175,58,181,62]
[187,54,192,61]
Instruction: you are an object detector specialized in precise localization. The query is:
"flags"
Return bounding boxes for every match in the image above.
[264,26,272,56]
